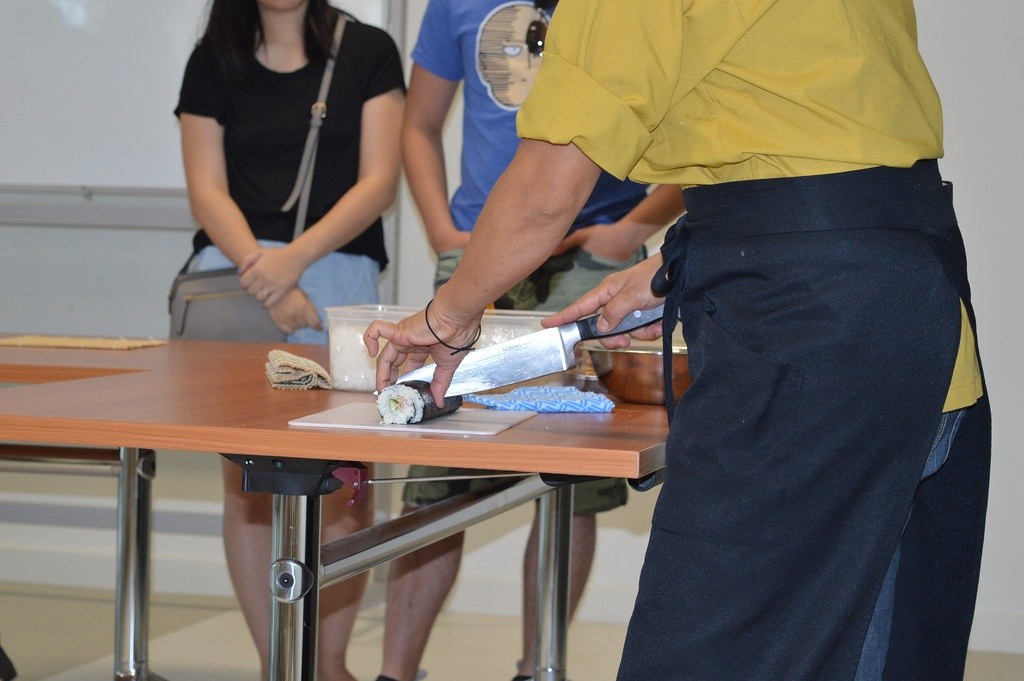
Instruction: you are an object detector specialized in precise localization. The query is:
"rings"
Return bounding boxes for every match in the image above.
[262,287,272,297]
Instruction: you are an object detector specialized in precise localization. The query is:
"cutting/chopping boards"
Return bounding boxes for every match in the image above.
[288,401,538,435]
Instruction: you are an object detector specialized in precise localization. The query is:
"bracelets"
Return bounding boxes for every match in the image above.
[425,299,481,356]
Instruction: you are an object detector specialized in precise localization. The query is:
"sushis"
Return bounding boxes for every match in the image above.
[376,379,462,424]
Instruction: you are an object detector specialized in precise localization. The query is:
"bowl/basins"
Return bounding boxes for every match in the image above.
[577,339,693,407]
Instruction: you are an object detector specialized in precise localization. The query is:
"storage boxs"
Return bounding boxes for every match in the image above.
[323,302,428,393]
[472,308,559,351]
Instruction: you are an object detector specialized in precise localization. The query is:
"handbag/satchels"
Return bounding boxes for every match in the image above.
[170,266,288,343]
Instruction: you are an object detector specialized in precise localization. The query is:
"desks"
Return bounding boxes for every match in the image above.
[0,332,668,681]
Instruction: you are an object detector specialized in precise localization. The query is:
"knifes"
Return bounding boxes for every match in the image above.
[372,301,683,400]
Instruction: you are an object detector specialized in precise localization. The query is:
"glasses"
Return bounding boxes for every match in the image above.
[526,0,559,55]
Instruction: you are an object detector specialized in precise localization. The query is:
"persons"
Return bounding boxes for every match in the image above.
[168,0,408,681]
[363,0,994,681]
